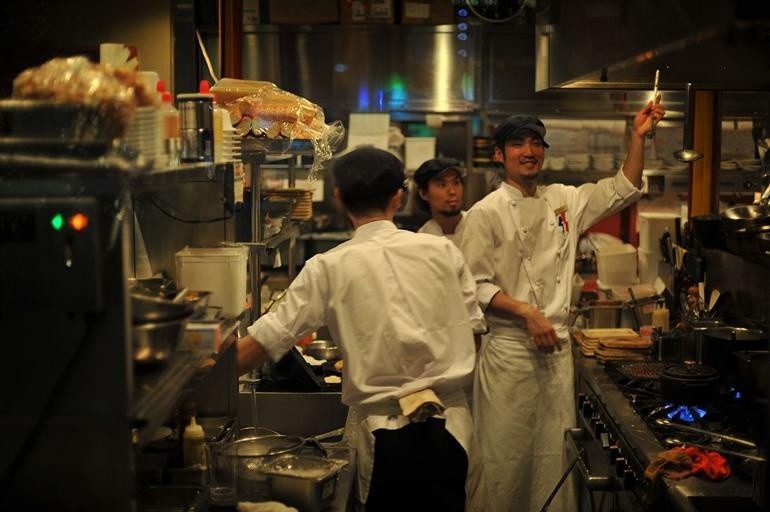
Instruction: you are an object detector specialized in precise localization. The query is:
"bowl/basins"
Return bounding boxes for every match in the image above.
[259,200,296,216]
[129,275,212,373]
[304,340,340,360]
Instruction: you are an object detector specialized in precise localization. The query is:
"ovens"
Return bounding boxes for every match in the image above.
[564,424,660,512]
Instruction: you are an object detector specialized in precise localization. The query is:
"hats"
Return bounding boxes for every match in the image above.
[492,113,547,145]
[412,157,468,187]
[330,146,408,190]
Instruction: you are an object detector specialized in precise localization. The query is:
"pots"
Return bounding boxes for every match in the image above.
[218,427,329,503]
[651,319,770,406]
[602,359,769,456]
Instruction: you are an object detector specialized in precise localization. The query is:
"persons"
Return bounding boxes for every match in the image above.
[238,146,486,512]
[459,92,666,512]
[413,157,467,249]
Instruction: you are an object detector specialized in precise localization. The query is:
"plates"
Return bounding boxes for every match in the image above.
[272,188,316,221]
[219,106,243,167]
[577,327,655,369]
[127,104,158,172]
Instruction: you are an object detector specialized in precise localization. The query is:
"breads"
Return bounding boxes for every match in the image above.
[15,55,160,138]
[211,78,323,139]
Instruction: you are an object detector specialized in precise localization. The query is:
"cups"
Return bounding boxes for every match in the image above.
[178,91,214,162]
[204,442,239,507]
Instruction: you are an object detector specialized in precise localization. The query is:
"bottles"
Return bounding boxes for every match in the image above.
[177,413,208,467]
[198,80,222,163]
[155,80,178,167]
[640,298,670,341]
